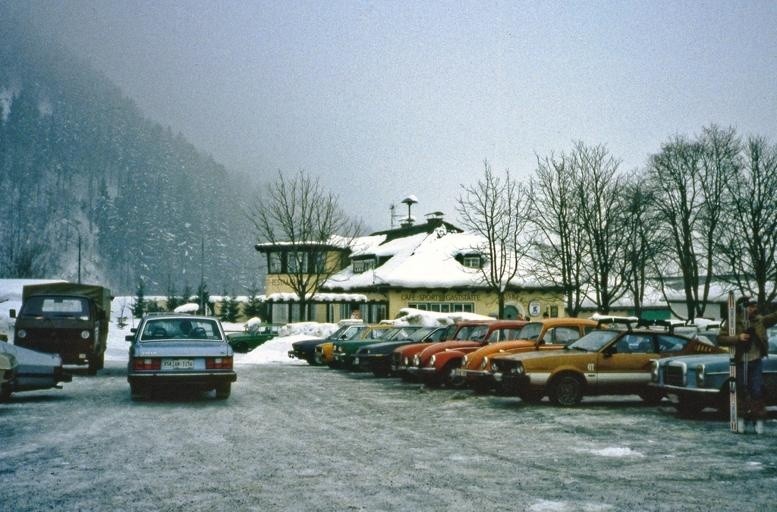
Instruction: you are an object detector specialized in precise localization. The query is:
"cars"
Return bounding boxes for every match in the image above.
[488,326,727,408]
[647,334,777,419]
[124,312,238,402]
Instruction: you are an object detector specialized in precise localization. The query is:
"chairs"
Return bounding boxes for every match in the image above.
[151,325,207,339]
[619,340,650,353]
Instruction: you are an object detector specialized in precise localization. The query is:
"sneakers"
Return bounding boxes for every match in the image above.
[754,419,764,434]
[738,417,745,434]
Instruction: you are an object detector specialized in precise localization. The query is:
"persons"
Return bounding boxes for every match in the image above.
[350,306,361,319]
[716,297,777,435]
[174,321,196,338]
[243,313,261,333]
[506,310,550,321]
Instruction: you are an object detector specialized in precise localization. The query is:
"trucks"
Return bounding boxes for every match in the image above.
[8,280,113,377]
